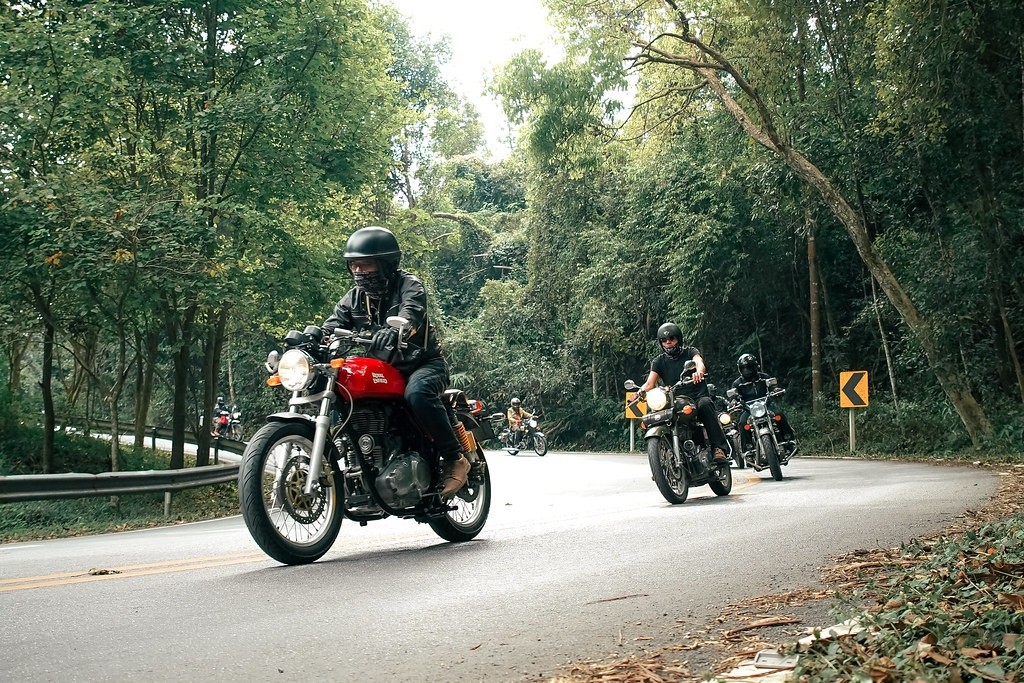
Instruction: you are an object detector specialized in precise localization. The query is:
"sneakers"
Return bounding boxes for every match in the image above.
[440,454,471,498]
[213,430,221,436]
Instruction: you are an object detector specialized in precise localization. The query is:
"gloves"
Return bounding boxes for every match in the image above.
[371,327,402,351]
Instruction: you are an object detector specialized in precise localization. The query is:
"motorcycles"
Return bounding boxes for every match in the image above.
[727,378,799,481]
[624,359,733,504]
[716,390,744,469]
[498,409,548,456]
[211,405,243,441]
[238,317,505,565]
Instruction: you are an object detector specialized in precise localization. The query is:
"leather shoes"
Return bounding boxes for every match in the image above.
[713,447,727,461]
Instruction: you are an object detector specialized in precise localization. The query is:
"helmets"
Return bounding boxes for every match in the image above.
[343,225,402,260]
[511,398,521,408]
[737,353,759,376]
[657,322,683,341]
[217,397,225,404]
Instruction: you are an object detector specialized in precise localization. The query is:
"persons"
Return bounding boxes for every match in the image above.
[629,323,731,481]
[212,397,234,436]
[706,383,733,419]
[507,398,533,447]
[282,226,471,497]
[727,354,795,457]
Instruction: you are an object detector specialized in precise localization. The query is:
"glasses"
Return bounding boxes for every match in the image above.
[660,337,677,342]
[347,260,381,272]
